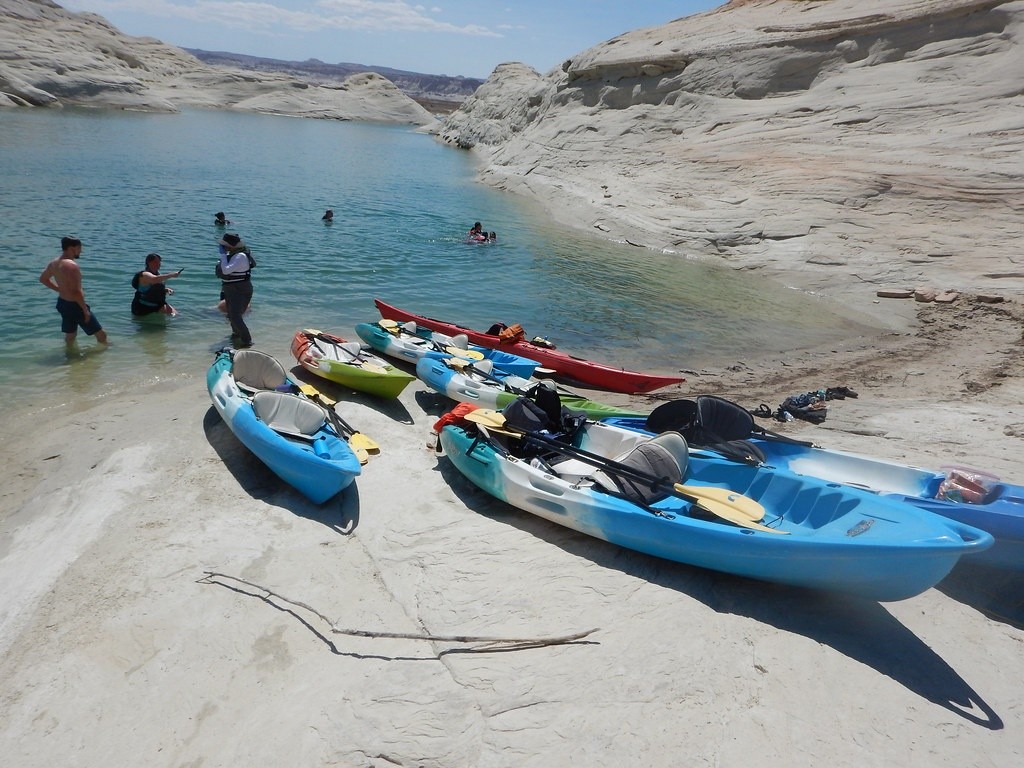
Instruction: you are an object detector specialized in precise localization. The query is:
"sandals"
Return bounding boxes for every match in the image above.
[773,410,787,422]
[750,404,771,417]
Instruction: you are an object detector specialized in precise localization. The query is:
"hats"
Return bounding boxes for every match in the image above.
[217,233,242,250]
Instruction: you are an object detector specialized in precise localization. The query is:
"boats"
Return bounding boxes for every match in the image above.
[288,329,416,402]
[372,299,688,395]
[433,402,997,603]
[592,388,1023,580]
[353,319,543,382]
[415,355,655,423]
[205,345,362,508]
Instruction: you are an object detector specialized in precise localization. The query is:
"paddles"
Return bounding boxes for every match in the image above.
[304,328,389,374]
[300,382,380,464]
[376,319,485,361]
[450,355,531,403]
[460,406,790,536]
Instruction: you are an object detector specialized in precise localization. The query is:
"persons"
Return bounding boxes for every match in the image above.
[470,222,497,243]
[40,236,107,344]
[216,233,257,346]
[322,210,334,220]
[131,253,181,316]
[214,212,230,225]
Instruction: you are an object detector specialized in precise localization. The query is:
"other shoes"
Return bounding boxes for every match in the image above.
[827,388,845,400]
[836,386,858,397]
